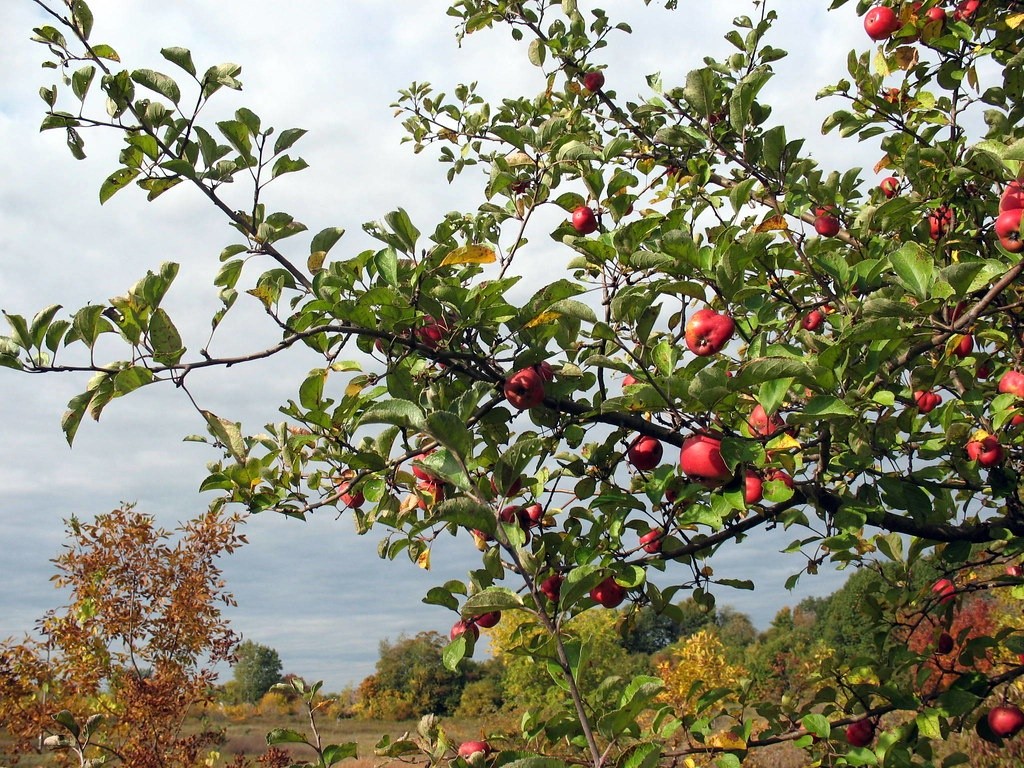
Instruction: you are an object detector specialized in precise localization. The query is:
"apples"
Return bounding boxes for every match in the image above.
[337,0,1024,768]
